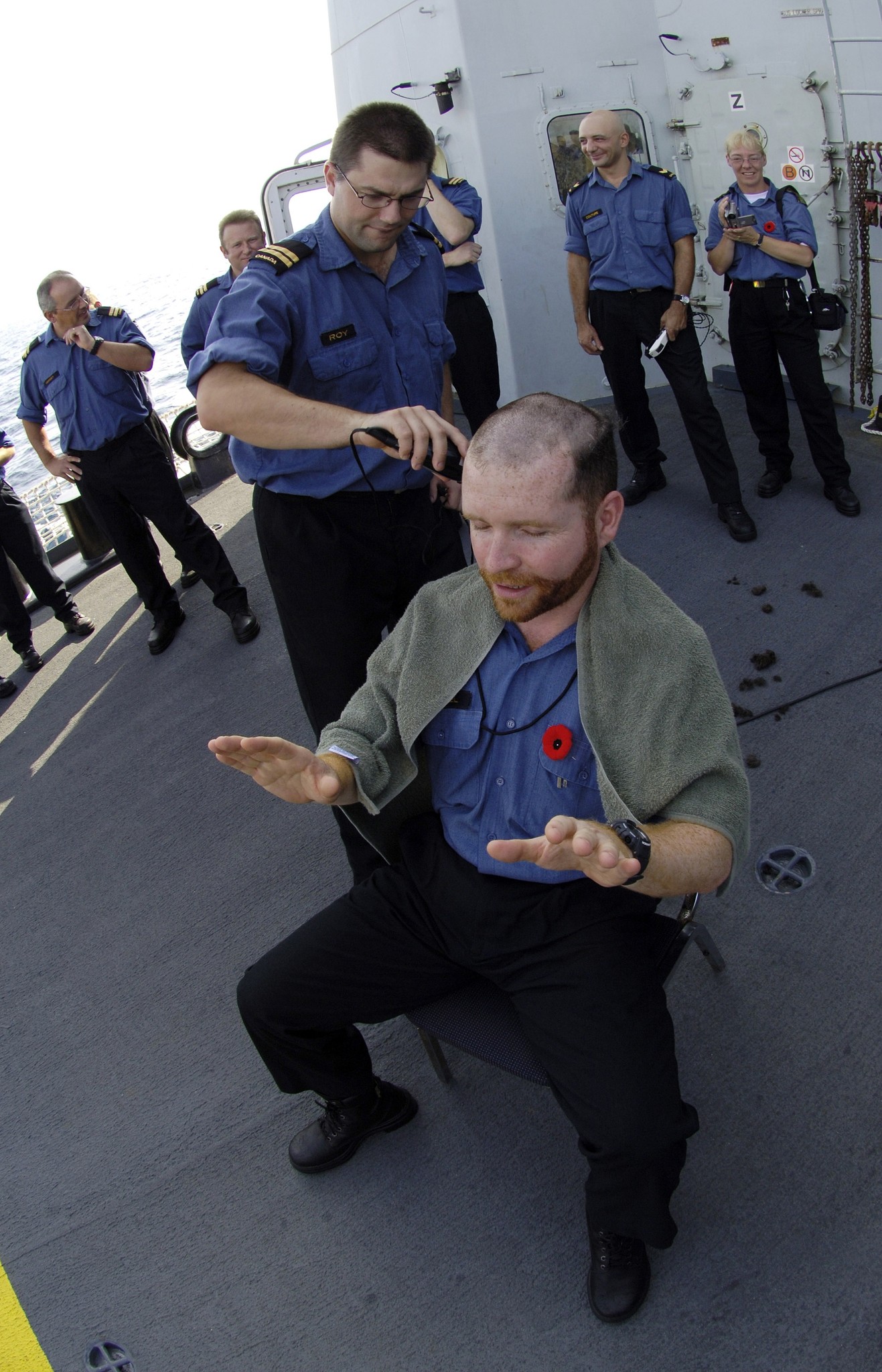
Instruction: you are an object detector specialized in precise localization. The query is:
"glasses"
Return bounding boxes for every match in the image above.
[53,287,91,312]
[335,163,434,210]
[727,154,763,164]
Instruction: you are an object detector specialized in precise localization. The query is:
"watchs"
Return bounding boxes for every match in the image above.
[90,336,104,357]
[672,295,691,306]
[607,819,651,886]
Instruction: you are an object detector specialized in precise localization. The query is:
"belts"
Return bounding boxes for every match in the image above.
[730,278,796,289]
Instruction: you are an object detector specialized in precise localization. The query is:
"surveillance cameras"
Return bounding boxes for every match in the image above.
[432,80,455,116]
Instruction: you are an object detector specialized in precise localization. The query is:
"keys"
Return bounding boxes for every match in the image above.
[785,300,791,312]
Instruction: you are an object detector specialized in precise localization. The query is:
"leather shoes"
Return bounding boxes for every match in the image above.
[824,484,860,515]
[584,1203,652,1321]
[757,466,792,498]
[717,498,758,541]
[19,646,43,672]
[64,612,95,636]
[148,606,185,655]
[619,463,668,506]
[0,676,16,699]
[227,604,260,643]
[180,562,200,588]
[289,1075,417,1174]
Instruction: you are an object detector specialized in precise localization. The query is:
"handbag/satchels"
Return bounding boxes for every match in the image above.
[807,289,848,332]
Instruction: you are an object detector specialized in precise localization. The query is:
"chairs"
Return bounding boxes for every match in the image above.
[403,890,727,1086]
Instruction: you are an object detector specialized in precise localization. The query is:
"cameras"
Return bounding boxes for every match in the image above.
[723,199,758,230]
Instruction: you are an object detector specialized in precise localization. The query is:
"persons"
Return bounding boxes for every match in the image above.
[703,127,862,518]
[403,125,503,441]
[16,270,263,655]
[204,386,756,1322]
[0,675,18,699]
[562,108,759,543]
[180,209,266,374]
[551,122,647,210]
[0,427,96,675]
[187,101,476,888]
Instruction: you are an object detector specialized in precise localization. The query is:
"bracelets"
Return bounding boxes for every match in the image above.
[755,233,764,250]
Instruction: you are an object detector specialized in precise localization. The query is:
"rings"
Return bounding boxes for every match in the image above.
[73,328,75,331]
[738,236,741,239]
